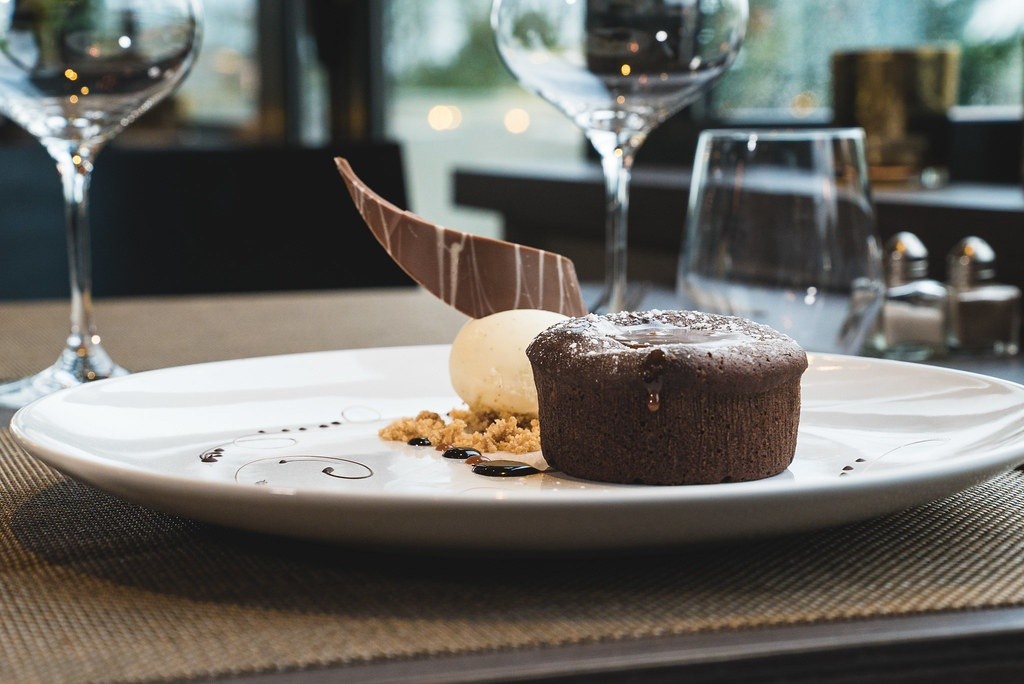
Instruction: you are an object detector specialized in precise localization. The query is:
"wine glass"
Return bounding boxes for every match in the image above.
[487,0,753,321]
[0,0,206,410]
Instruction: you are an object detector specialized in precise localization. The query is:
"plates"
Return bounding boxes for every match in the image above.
[10,341,1024,548]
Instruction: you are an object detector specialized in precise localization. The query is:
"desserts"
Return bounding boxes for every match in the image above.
[334,155,588,466]
[525,309,807,485]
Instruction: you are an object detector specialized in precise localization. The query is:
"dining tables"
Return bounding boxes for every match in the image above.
[1,283,1023,684]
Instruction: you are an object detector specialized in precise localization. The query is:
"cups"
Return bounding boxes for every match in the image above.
[680,127,887,357]
[829,44,957,180]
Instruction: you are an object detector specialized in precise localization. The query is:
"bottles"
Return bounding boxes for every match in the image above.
[950,237,1019,354]
[878,233,952,360]
